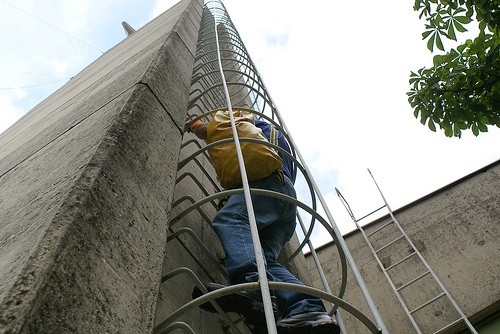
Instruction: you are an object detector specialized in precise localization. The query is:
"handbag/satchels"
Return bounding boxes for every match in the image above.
[206,104,283,189]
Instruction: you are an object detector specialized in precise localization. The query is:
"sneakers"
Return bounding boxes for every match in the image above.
[192,281,279,325]
[277,311,340,334]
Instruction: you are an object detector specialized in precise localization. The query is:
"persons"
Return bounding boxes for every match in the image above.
[188,104,341,334]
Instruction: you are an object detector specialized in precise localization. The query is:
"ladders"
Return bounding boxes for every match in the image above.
[335,167,481,334]
